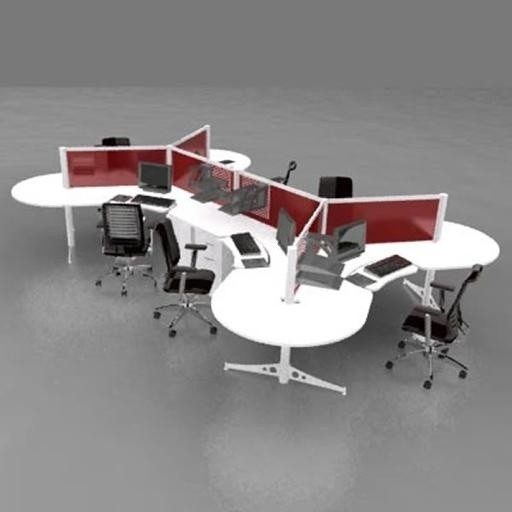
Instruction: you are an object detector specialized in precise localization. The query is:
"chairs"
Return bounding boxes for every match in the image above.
[152,218,219,335]
[92,202,157,298]
[272,159,296,184]
[102,137,130,146]
[318,176,353,199]
[385,265,484,389]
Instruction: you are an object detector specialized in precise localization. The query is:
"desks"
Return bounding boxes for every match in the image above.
[11,125,500,395]
